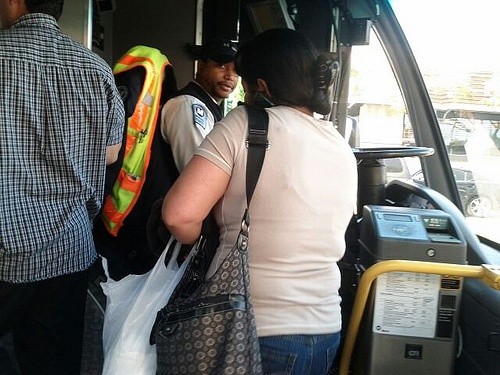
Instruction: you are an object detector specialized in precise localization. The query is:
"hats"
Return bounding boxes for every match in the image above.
[201,36,239,64]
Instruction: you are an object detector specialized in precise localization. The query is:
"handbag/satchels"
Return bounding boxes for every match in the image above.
[98,232,201,375]
[93,46,182,262]
[149,222,263,375]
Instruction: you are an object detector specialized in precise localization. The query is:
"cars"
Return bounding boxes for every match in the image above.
[410,167,492,218]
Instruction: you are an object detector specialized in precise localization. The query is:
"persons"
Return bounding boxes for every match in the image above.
[0,0,125,375]
[151,41,240,207]
[160,29,359,375]
